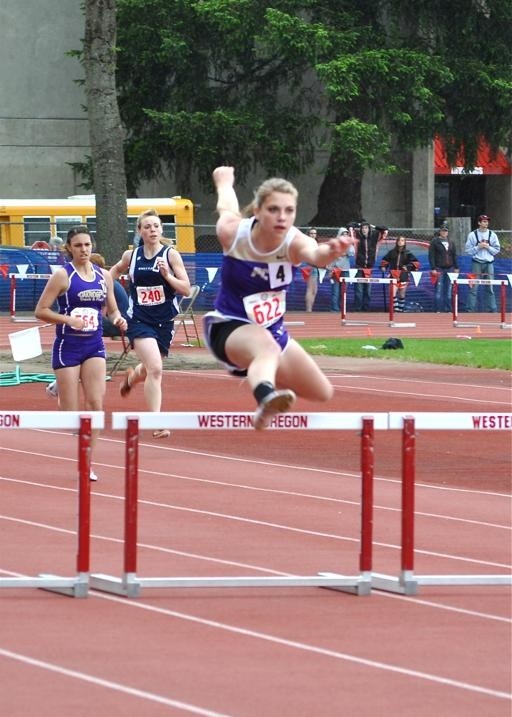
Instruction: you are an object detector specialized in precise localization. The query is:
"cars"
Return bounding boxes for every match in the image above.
[0,246,54,310]
[287,238,461,312]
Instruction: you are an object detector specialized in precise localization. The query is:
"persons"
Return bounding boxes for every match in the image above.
[427,224,459,314]
[108,207,191,439]
[353,221,381,312]
[464,214,501,313]
[31,224,129,482]
[328,226,356,313]
[45,252,133,400]
[297,227,319,312]
[199,164,361,432]
[381,235,420,312]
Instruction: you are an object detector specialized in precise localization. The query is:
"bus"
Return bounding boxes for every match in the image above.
[0,193,197,288]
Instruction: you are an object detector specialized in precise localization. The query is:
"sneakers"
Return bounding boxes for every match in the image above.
[254,388,296,432]
[153,430,171,439]
[45,379,60,398]
[119,367,135,398]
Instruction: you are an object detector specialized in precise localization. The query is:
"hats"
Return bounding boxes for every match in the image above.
[440,224,449,231]
[478,215,491,221]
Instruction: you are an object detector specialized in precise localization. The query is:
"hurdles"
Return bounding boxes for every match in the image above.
[0,409,106,599]
[117,275,128,286]
[338,276,397,327]
[90,410,389,598]
[9,272,53,323]
[452,278,509,328]
[371,412,511,596]
